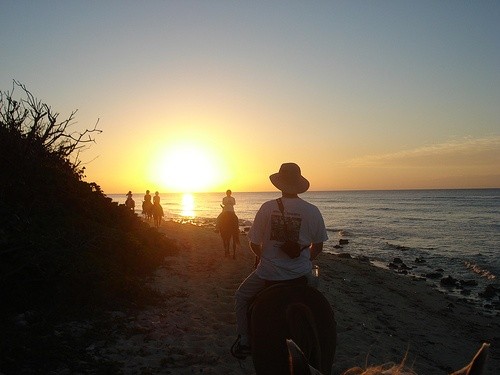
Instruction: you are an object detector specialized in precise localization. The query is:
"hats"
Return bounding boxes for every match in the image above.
[269,162,310,194]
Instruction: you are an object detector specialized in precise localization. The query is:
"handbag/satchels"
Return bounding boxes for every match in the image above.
[280,239,302,259]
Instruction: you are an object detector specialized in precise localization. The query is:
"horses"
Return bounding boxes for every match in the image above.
[125,198,135,212]
[219,204,240,259]
[141,201,164,228]
[248,240,338,375]
[287,339,491,375]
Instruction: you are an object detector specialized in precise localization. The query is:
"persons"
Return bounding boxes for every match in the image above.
[120,190,165,217]
[213,187,242,232]
[235,163,328,352]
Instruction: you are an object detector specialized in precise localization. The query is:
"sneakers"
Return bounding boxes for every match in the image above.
[234,340,250,353]
